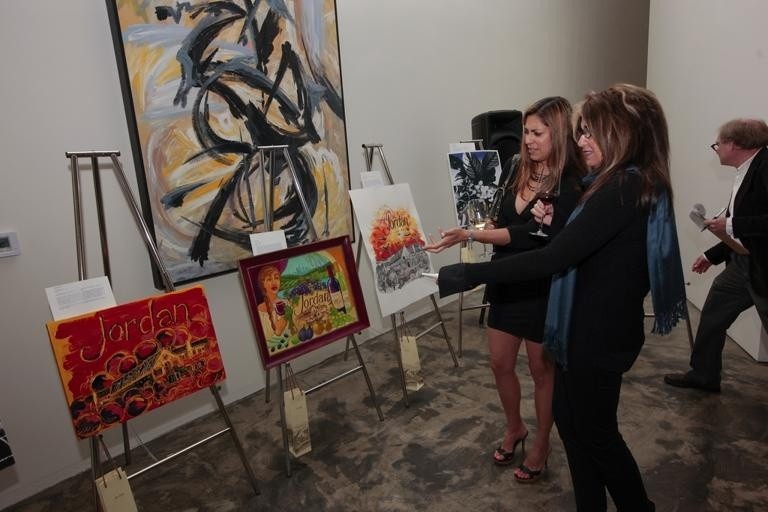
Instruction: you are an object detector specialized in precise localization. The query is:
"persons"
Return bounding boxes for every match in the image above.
[435,82,673,511]
[252,266,295,349]
[662,118,768,396]
[421,96,586,484]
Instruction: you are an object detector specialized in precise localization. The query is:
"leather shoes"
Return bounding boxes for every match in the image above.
[664,369,721,396]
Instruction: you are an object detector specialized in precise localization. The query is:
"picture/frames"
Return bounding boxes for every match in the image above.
[104,0,358,292]
[236,236,371,369]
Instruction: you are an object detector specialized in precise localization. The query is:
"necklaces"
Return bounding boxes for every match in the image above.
[529,172,549,183]
[526,182,540,192]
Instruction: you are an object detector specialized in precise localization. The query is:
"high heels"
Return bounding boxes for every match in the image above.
[513,442,553,482]
[492,427,530,467]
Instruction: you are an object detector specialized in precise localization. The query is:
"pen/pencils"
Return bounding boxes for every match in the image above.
[700,207,727,232]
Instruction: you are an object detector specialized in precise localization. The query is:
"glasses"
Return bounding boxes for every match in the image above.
[578,124,593,139]
[708,141,732,153]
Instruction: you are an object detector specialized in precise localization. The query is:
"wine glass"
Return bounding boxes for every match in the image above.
[467,206,497,259]
[528,173,557,237]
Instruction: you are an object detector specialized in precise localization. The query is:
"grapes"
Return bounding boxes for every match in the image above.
[331,310,352,327]
[291,280,321,295]
[295,326,313,342]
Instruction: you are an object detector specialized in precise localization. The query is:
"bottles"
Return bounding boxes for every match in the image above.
[326,265,344,315]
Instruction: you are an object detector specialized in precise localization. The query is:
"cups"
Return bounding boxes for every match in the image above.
[275,301,288,319]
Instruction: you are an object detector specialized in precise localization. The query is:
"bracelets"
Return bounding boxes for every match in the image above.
[467,229,474,244]
